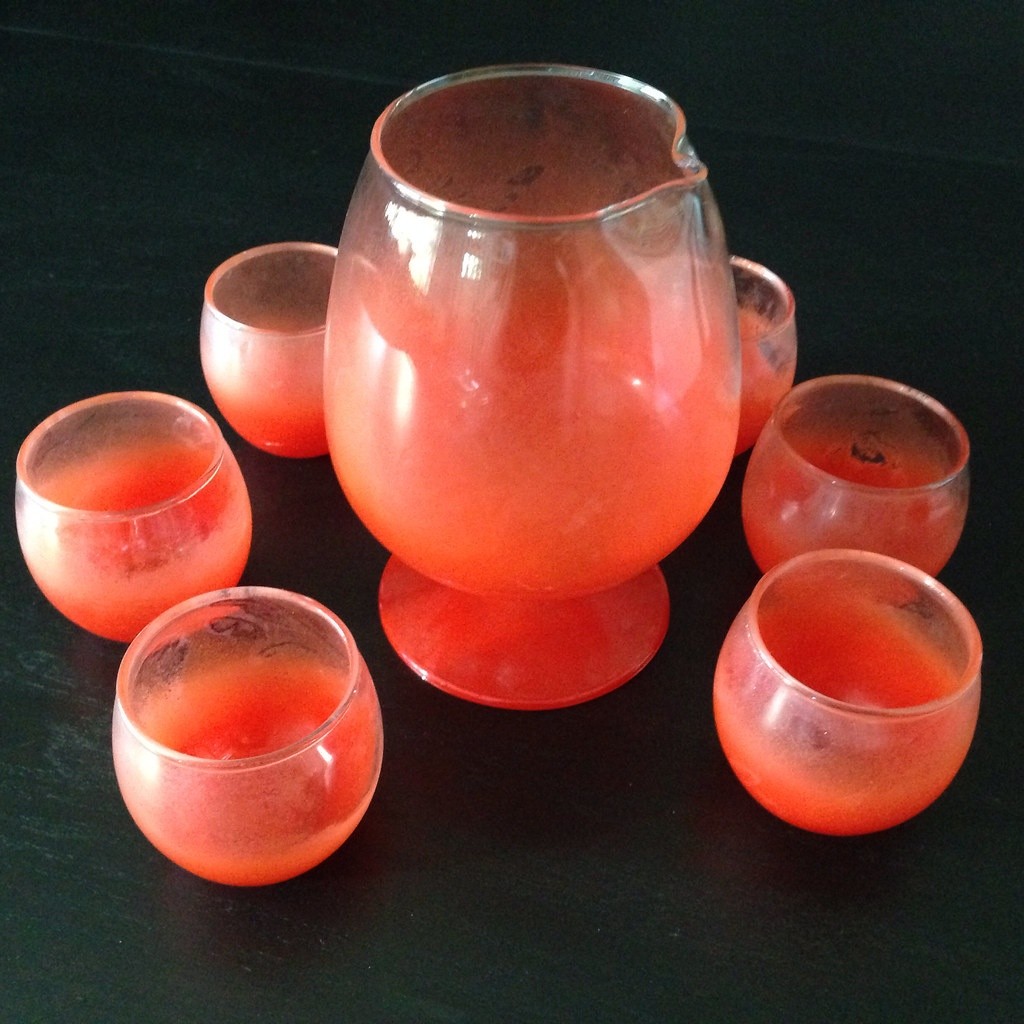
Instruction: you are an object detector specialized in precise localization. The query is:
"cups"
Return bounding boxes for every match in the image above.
[110,587,387,886]
[198,242,384,457]
[14,391,253,640]
[742,373,969,575]
[711,547,983,838]
[731,254,798,460]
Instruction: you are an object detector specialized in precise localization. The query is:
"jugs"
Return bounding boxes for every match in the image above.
[321,66,743,710]
